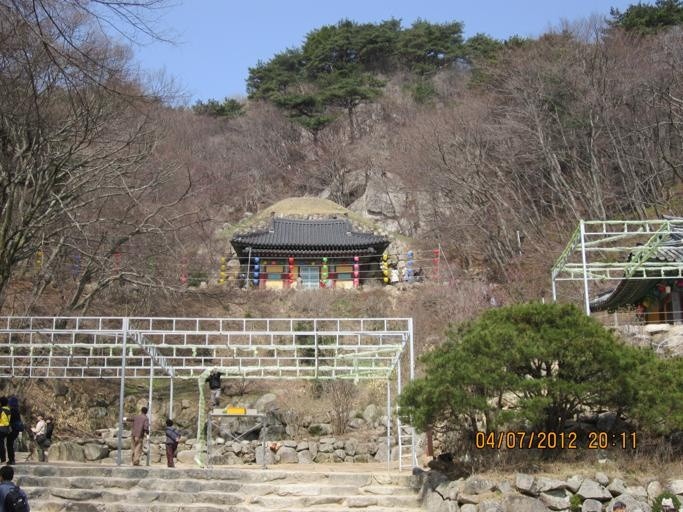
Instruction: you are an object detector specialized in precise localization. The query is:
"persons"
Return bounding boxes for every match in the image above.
[205,367,222,407]
[123,406,150,467]
[0,466,30,511]
[0,395,24,465]
[165,418,182,468]
[29,412,55,463]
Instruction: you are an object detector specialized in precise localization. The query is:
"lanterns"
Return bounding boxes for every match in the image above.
[179,254,188,283]
[252,241,448,286]
[637,280,683,313]
[32,249,43,276]
[219,257,227,285]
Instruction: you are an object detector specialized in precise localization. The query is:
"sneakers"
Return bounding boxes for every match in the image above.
[0,460,15,465]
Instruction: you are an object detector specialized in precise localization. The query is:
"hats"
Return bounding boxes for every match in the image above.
[9,397,17,407]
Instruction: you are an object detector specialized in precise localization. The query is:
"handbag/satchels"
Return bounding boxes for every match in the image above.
[14,420,24,431]
[36,434,45,444]
[5,488,27,512]
